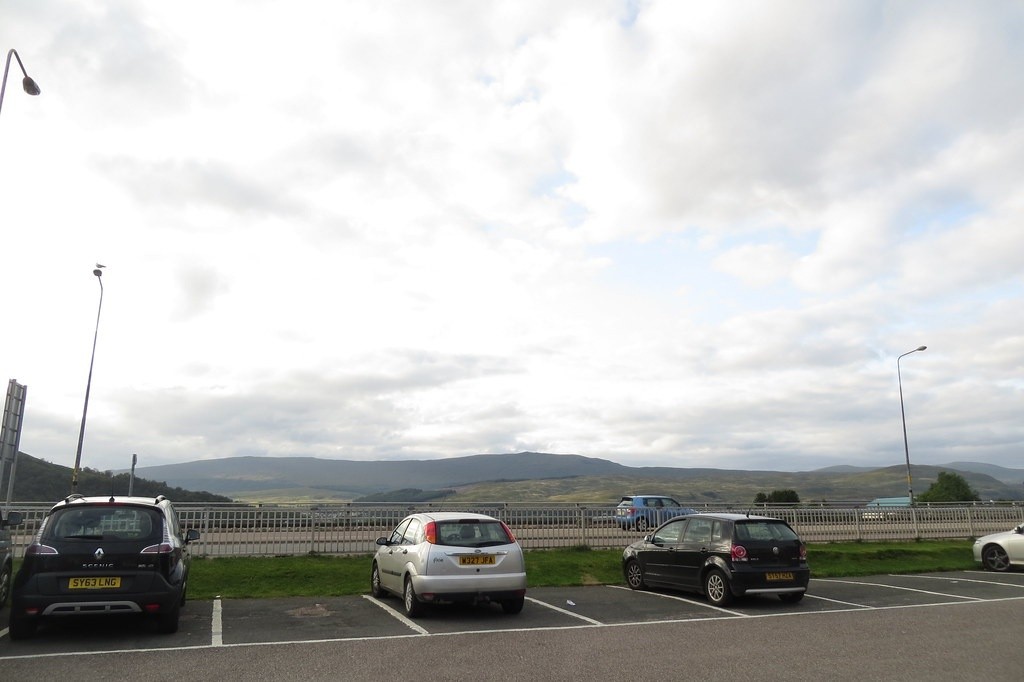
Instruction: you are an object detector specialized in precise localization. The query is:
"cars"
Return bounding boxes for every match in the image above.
[972,521,1024,572]
[0,504,24,611]
[865,497,918,521]
[371,512,528,619]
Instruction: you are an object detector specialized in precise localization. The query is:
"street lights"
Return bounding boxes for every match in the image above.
[896,345,927,541]
[0,44,41,119]
[68,268,105,497]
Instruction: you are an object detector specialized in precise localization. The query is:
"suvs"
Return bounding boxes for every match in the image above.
[622,512,811,606]
[7,494,201,642]
[617,496,702,533]
